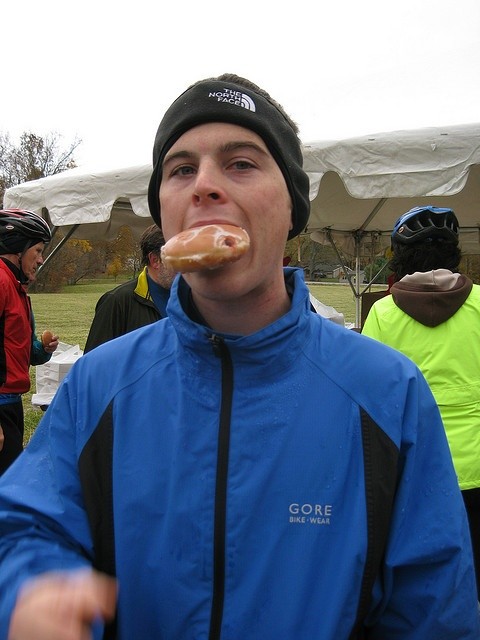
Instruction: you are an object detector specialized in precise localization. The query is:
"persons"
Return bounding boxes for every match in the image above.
[359,203,480,599]
[0,205,60,474]
[0,73,480,639]
[82,222,177,355]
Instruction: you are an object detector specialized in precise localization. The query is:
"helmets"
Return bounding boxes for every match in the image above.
[1,209,53,243]
[391,205,461,244]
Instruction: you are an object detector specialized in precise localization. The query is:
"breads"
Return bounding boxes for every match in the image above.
[161,225,250,273]
